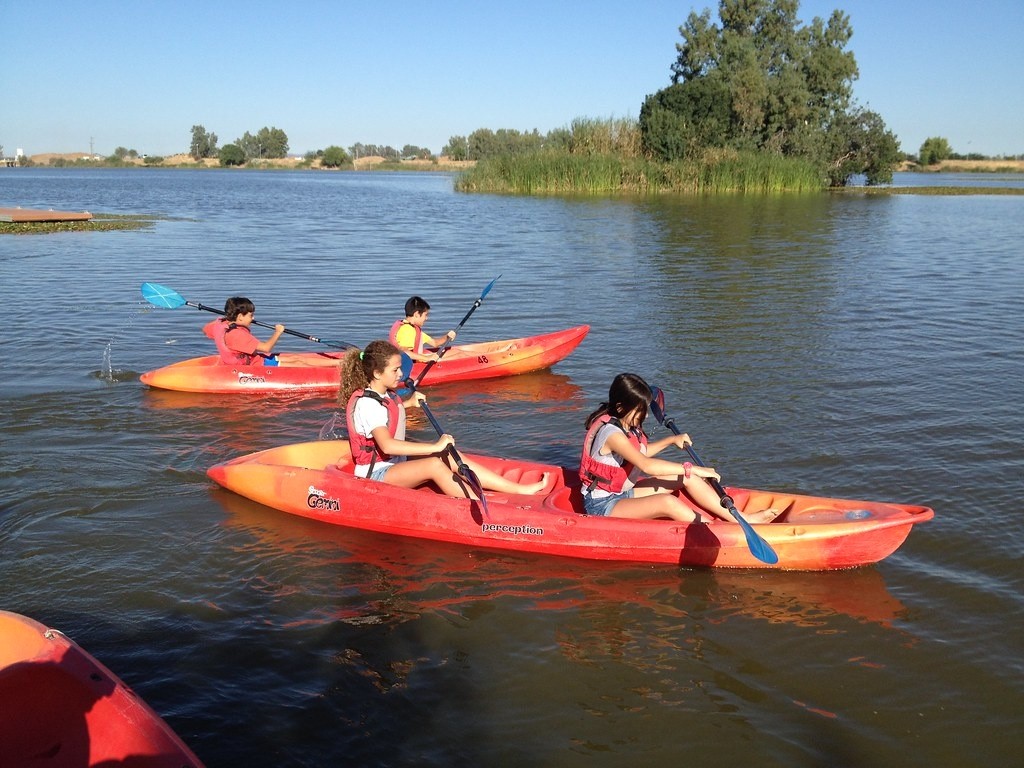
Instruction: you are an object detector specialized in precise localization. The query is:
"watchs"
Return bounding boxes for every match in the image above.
[683,462,693,479]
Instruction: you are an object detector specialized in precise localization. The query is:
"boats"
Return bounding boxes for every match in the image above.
[205,439,933,572]
[1,610,202,768]
[137,326,595,397]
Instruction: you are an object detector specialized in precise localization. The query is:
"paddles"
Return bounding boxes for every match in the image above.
[141,282,360,351]
[649,386,778,565]
[391,274,503,399]
[398,350,491,518]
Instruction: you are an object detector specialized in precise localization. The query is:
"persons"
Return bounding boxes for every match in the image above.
[336,340,551,511]
[578,373,779,525]
[387,295,457,363]
[200,296,349,367]
